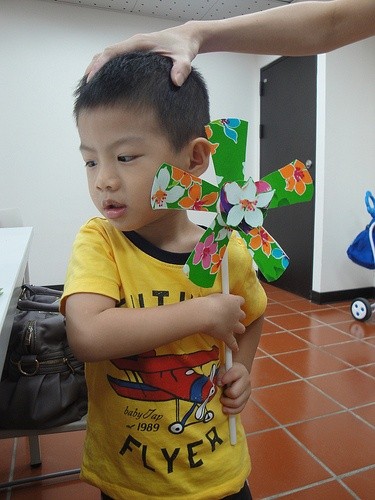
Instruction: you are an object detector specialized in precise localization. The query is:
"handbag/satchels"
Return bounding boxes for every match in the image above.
[1,284,91,429]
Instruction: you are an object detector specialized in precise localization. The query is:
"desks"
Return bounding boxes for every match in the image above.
[0,227,44,471]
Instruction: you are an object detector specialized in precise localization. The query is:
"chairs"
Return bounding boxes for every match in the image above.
[0,415,100,491]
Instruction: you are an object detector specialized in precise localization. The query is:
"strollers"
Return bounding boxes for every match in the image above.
[347,190,375,322]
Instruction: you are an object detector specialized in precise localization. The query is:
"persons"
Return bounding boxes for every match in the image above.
[83,1,375,87]
[50,53,269,497]
